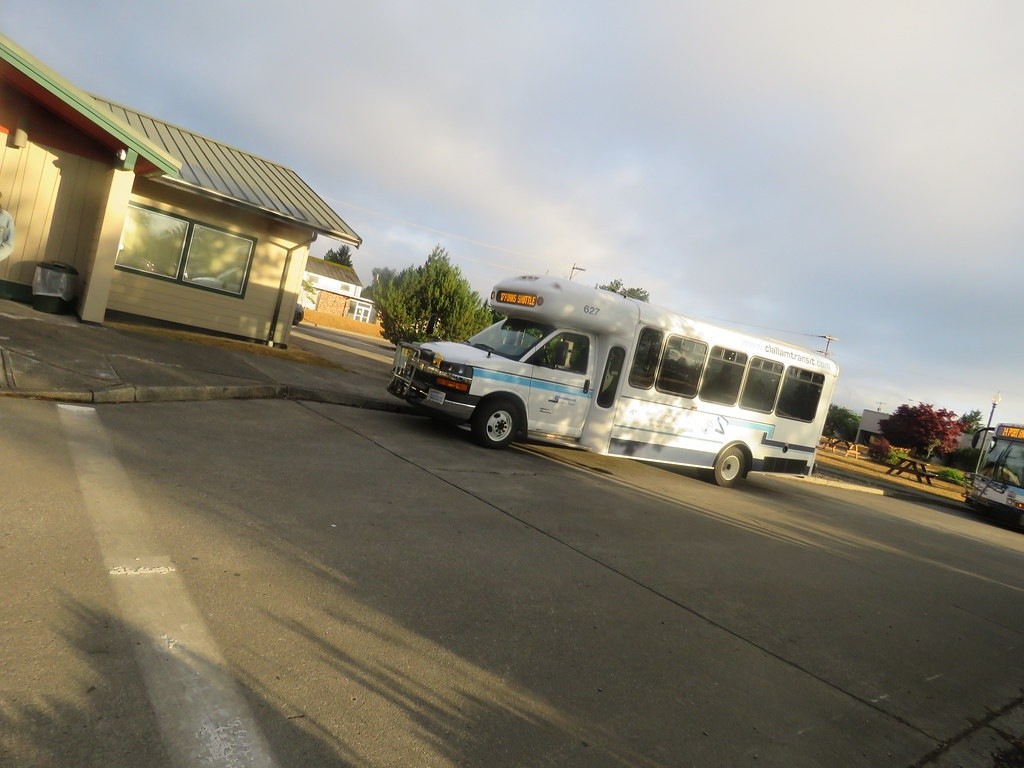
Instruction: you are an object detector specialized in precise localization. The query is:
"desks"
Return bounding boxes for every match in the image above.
[823,436,860,459]
[885,456,932,486]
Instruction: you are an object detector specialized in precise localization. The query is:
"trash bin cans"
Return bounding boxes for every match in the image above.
[30,260,80,315]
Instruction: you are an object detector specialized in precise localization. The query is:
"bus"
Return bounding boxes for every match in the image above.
[189,262,246,293]
[961,424,1024,517]
[385,271,840,488]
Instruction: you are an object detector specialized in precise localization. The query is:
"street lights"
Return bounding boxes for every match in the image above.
[569,268,586,281]
[970,394,1001,473]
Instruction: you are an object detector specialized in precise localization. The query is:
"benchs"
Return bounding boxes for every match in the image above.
[885,462,895,468]
[925,472,939,479]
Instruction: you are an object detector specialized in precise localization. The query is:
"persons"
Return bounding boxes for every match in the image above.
[0,191,15,262]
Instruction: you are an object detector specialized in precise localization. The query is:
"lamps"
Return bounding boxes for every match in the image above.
[13,114,32,148]
